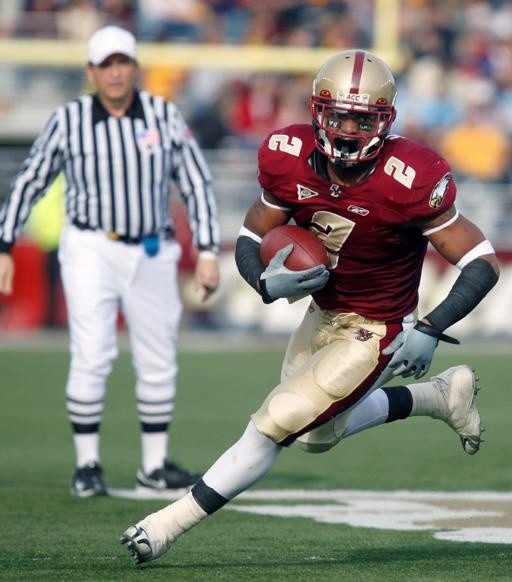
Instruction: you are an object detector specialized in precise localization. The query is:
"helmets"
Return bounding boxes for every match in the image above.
[306,49,398,182]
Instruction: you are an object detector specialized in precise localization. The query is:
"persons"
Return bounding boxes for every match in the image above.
[0,25,224,499]
[119,51,502,565]
[1,0,512,340]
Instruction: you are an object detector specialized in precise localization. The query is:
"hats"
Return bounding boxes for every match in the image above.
[87,24,139,66]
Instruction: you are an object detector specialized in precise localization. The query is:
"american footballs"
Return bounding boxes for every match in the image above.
[260,225,329,271]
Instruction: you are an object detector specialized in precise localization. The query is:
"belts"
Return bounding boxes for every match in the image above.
[73,218,145,246]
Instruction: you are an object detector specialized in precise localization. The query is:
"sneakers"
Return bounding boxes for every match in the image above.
[136,459,205,497]
[433,363,484,454]
[120,512,178,566]
[69,461,109,499]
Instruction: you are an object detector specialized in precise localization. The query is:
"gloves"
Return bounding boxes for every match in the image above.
[383,320,440,380]
[258,244,329,305]
